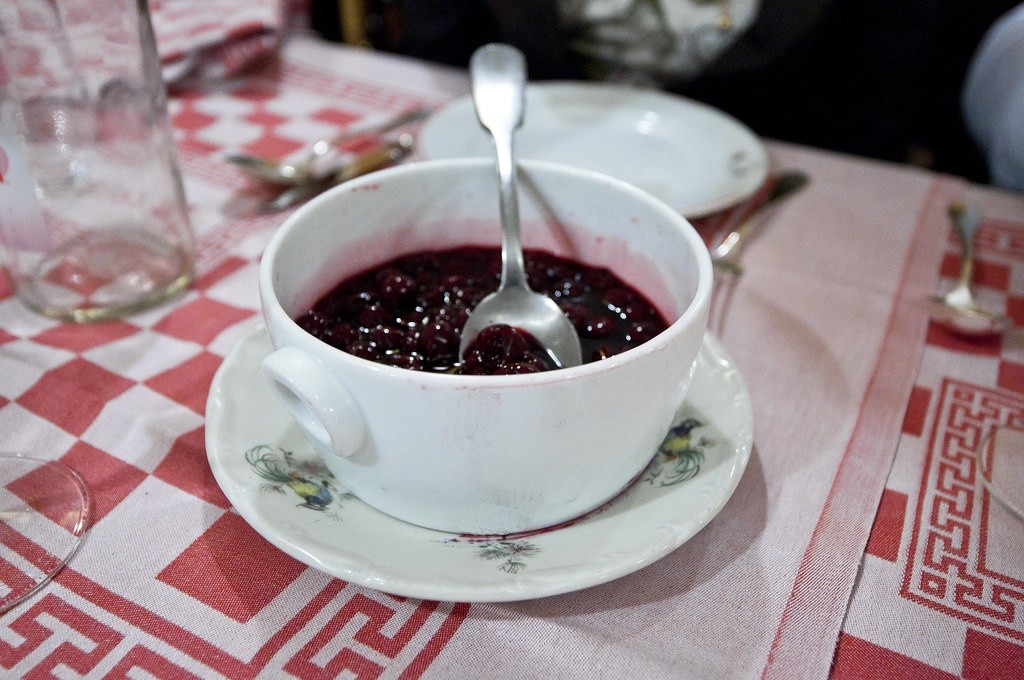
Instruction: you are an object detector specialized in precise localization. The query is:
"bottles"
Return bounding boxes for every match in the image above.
[0,0,199,325]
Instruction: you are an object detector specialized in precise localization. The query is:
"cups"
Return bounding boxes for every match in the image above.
[255,156,719,537]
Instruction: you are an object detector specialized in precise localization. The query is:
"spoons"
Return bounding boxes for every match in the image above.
[925,205,1014,339]
[457,44,585,372]
[225,99,436,188]
[707,166,813,273]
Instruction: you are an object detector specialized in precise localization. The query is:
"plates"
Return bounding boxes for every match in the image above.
[203,323,762,608]
[415,82,768,218]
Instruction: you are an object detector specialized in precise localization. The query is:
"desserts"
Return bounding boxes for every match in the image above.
[290,244,673,373]
